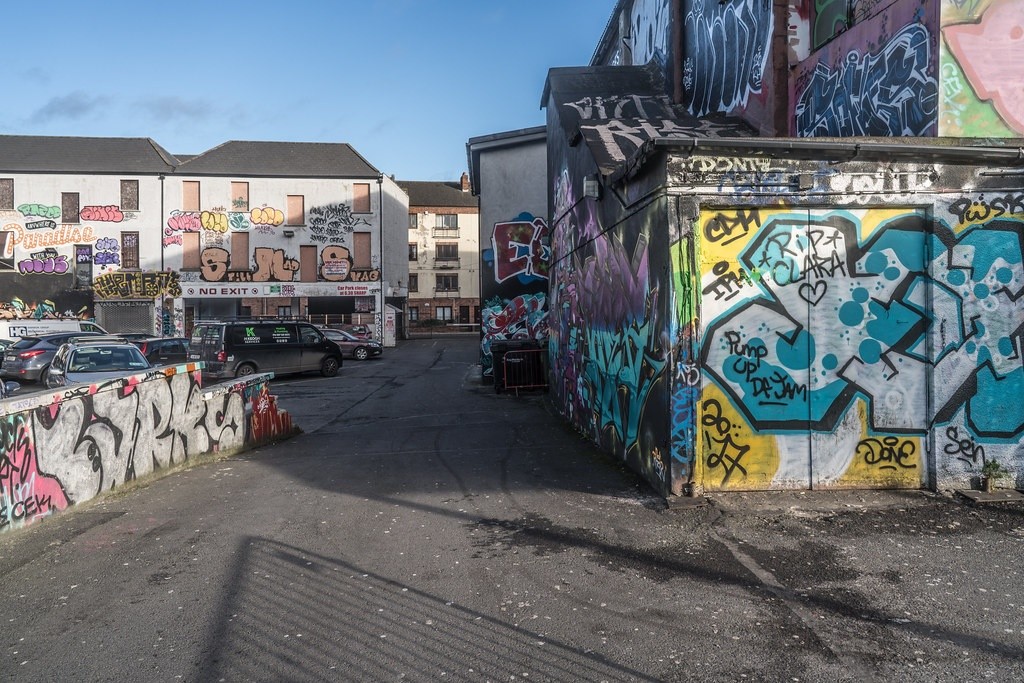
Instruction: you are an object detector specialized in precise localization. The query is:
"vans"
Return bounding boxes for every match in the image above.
[190,315,344,382]
[1,317,110,344]
[130,339,191,368]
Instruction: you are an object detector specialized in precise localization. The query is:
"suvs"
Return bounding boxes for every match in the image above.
[47,338,152,387]
[3,331,109,391]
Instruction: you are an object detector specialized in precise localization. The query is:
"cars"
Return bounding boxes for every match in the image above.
[317,329,384,361]
[341,324,372,339]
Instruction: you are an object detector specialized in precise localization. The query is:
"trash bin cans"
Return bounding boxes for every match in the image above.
[491,339,541,394]
